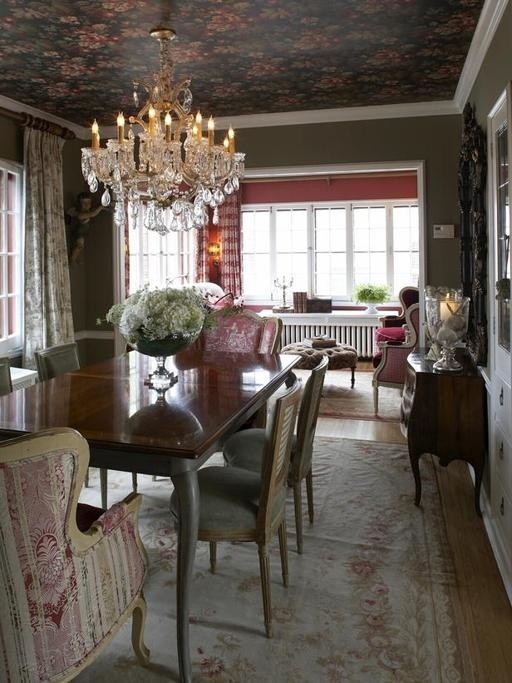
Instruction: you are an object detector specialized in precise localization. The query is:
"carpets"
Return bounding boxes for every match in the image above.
[267,367,406,425]
[72,434,474,682]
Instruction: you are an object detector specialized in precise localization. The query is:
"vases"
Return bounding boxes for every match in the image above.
[118,333,200,383]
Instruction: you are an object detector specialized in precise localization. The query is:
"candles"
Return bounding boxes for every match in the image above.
[438,292,456,322]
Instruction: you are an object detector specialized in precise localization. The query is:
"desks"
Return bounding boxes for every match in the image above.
[0,348,304,681]
[401,344,488,519]
[8,365,39,393]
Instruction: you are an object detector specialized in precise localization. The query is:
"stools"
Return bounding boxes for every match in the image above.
[280,336,357,389]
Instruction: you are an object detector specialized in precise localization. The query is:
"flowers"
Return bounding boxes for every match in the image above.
[96,281,216,360]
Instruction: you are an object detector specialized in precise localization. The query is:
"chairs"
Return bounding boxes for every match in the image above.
[189,306,285,454]
[33,341,138,509]
[0,355,14,398]
[223,355,328,556]
[179,280,245,310]
[0,425,154,681]
[168,378,304,638]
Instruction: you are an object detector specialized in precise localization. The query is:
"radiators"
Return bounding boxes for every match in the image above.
[257,308,400,358]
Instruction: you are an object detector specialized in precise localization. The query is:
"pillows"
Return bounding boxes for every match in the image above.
[203,290,234,309]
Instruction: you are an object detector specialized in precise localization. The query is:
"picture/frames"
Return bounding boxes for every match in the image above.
[454,99,489,368]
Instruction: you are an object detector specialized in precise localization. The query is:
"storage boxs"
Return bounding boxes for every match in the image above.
[306,295,333,313]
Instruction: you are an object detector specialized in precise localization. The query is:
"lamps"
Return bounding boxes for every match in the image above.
[77,28,248,230]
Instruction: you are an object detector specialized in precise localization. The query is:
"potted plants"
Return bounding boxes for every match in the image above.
[352,282,393,314]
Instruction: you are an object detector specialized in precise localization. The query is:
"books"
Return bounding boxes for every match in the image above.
[292,291,308,312]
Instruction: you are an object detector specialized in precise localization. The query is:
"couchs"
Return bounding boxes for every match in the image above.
[370,285,420,414]
